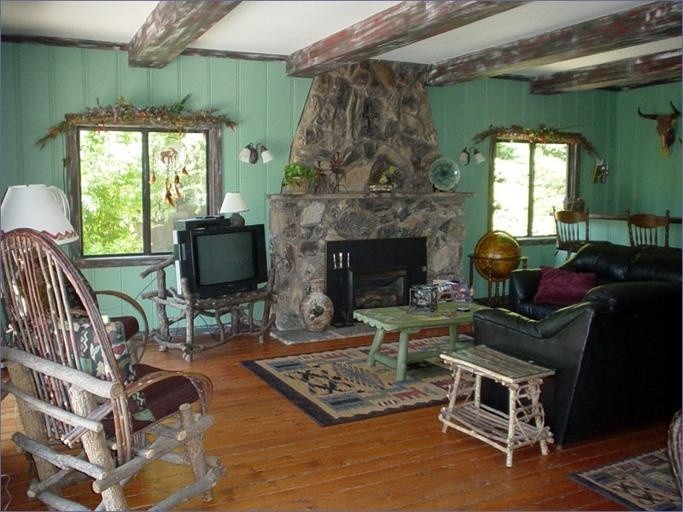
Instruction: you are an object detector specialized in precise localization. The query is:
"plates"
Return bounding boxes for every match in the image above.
[427,157,461,192]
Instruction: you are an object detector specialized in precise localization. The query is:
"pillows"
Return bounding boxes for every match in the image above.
[25,315,160,432]
[534,267,598,307]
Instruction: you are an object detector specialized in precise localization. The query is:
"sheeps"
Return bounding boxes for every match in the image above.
[638,100,682,155]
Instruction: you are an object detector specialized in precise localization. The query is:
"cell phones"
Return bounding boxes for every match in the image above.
[457,308,471,311]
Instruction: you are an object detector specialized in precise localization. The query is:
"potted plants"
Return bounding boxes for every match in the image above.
[366,155,401,192]
[285,161,315,198]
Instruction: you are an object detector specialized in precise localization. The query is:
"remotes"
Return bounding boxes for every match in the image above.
[204,215,224,219]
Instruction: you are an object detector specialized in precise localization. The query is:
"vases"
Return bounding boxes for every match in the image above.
[299,279,336,331]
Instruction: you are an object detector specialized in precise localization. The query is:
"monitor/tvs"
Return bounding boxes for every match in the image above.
[177,224,267,298]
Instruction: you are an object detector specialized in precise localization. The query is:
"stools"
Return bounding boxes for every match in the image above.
[437,341,553,465]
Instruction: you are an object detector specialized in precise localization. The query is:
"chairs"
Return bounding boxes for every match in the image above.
[552,205,593,252]
[624,211,670,245]
[0,229,229,512]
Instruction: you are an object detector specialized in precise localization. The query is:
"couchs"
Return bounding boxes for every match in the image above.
[469,280,680,446]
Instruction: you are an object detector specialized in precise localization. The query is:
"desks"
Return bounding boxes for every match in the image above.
[139,253,277,362]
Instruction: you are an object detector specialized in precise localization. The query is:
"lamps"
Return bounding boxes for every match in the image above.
[236,139,275,171]
[221,193,249,228]
[457,146,485,166]
[0,178,82,251]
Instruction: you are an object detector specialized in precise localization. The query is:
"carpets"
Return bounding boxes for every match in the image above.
[566,446,682,510]
[241,337,483,426]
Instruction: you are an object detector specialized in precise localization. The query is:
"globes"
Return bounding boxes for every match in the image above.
[466,230,528,308]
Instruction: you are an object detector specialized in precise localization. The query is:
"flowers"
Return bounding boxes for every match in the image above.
[33,92,237,154]
[467,123,599,162]
